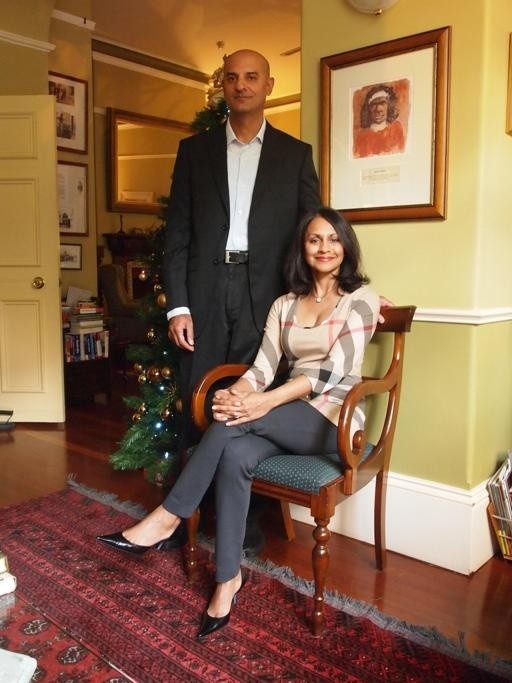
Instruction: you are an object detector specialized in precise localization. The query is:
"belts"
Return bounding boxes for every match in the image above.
[211,249,248,268]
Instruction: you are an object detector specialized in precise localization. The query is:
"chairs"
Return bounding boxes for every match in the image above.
[183,306,416,638]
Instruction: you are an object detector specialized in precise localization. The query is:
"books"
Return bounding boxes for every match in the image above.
[486,448,512,560]
[60,285,109,364]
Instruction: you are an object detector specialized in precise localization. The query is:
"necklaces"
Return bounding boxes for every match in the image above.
[315,297,322,303]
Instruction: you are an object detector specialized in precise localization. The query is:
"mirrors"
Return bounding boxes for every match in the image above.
[105,106,204,215]
[118,154,175,201]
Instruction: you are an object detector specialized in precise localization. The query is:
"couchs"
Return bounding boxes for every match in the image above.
[99,264,170,346]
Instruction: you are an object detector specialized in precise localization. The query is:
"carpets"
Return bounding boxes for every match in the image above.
[0,475,512,683]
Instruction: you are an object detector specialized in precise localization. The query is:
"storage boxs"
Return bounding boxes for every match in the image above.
[66,367,81,402]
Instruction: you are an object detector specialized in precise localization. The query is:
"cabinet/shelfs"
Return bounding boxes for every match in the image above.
[102,232,156,299]
[65,358,113,405]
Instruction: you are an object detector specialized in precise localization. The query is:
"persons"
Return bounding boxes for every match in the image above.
[152,48,322,560]
[97,208,380,639]
[354,85,406,157]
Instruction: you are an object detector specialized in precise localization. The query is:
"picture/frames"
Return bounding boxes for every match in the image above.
[57,160,90,237]
[319,24,452,222]
[47,71,89,155]
[60,243,82,270]
[121,190,154,203]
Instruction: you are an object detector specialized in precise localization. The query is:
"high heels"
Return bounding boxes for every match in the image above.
[98,524,185,558]
[198,568,243,639]
